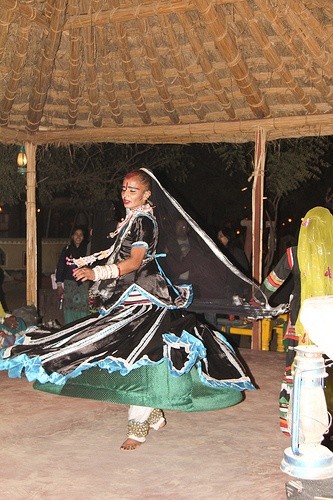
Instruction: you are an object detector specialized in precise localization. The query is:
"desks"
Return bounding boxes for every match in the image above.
[188,300,278,350]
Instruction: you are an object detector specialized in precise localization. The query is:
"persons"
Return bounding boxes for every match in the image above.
[56,220,109,326]
[67,168,169,451]
[161,217,251,301]
[250,206,333,451]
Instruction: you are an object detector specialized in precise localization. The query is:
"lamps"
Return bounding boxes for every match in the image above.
[16,146,27,175]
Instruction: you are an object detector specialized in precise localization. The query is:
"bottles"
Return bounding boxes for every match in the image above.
[57,294,64,311]
